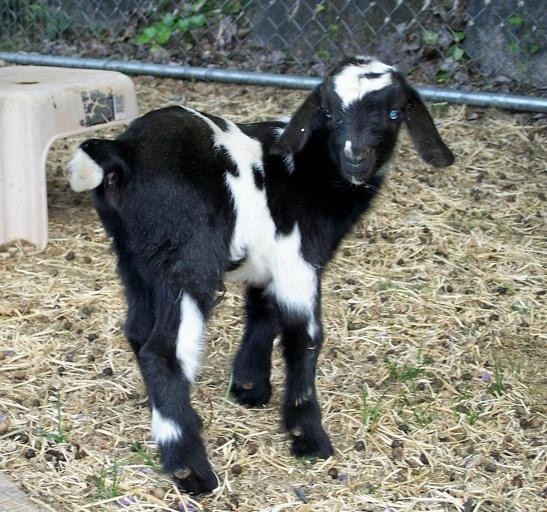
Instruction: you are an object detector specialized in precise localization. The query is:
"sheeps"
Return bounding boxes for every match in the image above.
[59,54,455,498]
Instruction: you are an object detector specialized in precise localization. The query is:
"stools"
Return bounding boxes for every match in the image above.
[0,64,138,252]
